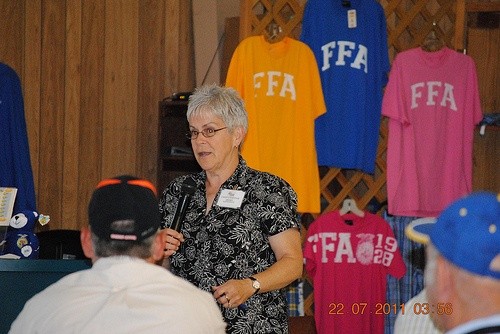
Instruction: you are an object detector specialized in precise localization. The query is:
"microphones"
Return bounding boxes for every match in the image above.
[162,177,196,250]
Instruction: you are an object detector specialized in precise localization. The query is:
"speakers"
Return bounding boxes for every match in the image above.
[155,93,203,174]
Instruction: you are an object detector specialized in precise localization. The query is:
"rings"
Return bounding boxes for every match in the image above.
[225,294,230,302]
[228,302,231,307]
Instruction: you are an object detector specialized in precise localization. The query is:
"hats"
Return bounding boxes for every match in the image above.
[88,175,160,242]
[405,193,500,279]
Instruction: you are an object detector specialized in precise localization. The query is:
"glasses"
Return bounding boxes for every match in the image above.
[185,126,231,139]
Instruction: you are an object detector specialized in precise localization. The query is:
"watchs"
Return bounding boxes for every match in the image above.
[248,276,261,296]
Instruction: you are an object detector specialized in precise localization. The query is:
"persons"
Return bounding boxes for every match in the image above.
[404,190,500,334]
[157,83,304,334]
[7,174,227,334]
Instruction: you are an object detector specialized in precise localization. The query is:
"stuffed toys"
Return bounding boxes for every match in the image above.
[5,208,40,260]
